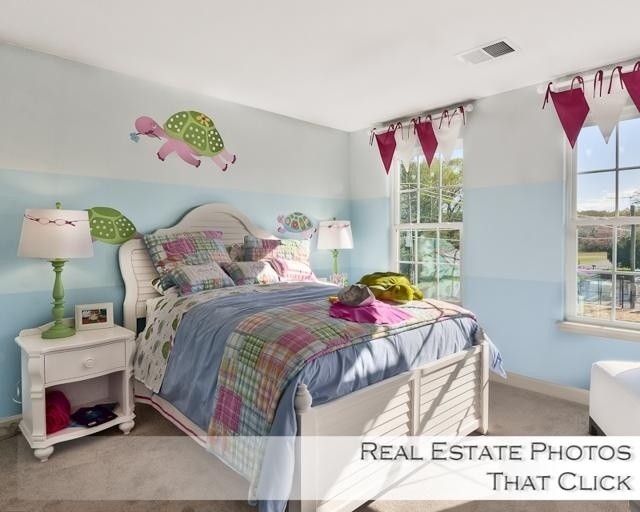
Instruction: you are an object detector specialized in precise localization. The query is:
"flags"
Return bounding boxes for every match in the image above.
[373,129,396,176]
[620,70,640,114]
[413,121,437,168]
[548,86,590,150]
[578,75,629,146]
[393,127,417,173]
[431,114,464,164]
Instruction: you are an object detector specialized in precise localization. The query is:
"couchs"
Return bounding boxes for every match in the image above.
[588,362,639,438]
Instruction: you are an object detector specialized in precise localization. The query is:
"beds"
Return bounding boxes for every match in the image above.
[120,201,507,512]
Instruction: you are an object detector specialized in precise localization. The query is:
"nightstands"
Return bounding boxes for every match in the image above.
[17,320,132,462]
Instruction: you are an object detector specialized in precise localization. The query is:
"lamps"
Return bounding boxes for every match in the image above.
[17,209,94,339]
[318,221,355,285]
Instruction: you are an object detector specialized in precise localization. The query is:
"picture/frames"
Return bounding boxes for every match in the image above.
[76,304,115,330]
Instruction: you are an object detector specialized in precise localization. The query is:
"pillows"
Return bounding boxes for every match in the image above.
[221,261,278,288]
[244,235,310,261]
[270,259,316,284]
[163,252,233,295]
[151,277,179,295]
[144,230,231,271]
